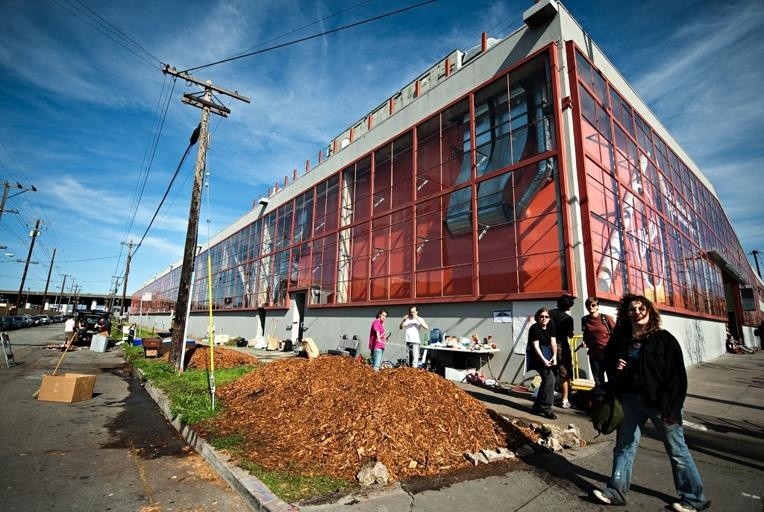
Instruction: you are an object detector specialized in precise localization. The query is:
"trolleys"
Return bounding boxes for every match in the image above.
[569,334,596,409]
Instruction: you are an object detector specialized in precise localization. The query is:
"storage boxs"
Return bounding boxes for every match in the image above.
[37,373,96,403]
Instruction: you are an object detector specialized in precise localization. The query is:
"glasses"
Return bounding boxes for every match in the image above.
[539,315,549,318]
[588,304,597,307]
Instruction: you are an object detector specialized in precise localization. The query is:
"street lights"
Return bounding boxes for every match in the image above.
[24,286,31,308]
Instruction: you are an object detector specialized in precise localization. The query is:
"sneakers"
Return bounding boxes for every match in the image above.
[592,489,612,504]
[561,400,571,409]
[544,412,557,419]
[672,501,701,512]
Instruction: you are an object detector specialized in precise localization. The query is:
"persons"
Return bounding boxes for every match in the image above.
[94,316,105,335]
[72,315,84,341]
[61,314,75,347]
[590,291,713,512]
[368,308,391,373]
[524,306,558,420]
[397,304,429,369]
[726,334,758,354]
[754,320,763,351]
[580,297,617,393]
[548,294,575,409]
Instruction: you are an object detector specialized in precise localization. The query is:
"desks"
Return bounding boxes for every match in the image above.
[421,345,500,374]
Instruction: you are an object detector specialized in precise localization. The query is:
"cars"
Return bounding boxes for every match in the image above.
[0,314,72,331]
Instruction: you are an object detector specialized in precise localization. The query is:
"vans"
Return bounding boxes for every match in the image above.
[72,308,115,344]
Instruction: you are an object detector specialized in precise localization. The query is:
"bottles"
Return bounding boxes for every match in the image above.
[424,332,429,346]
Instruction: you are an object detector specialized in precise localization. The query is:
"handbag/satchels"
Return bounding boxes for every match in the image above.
[587,381,626,440]
